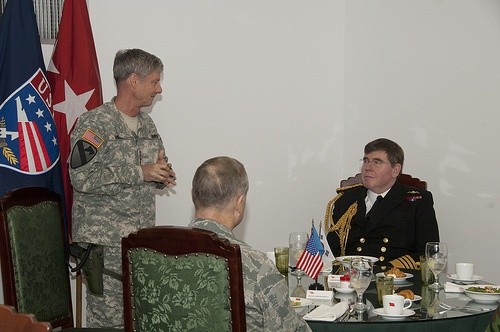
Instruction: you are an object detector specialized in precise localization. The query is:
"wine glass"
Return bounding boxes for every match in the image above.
[349,257,373,310]
[289,231,311,274]
[427,286,445,319]
[350,307,369,322]
[424,242,448,289]
[290,274,307,299]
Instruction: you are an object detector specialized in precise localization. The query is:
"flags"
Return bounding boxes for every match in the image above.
[48,0,103,245]
[0,0,67,239]
[319,228,336,268]
[294,229,322,281]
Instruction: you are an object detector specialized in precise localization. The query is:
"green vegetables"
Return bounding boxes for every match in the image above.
[465,286,500,293]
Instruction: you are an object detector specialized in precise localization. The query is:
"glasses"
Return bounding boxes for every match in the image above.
[360,158,393,169]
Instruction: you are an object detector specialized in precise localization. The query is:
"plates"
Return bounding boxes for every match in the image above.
[405,301,423,310]
[446,274,483,284]
[334,291,358,299]
[373,307,416,320]
[334,281,358,294]
[376,271,414,279]
[396,295,422,302]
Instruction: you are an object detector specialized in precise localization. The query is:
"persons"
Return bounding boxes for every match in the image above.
[67,48,177,329]
[323,138,440,268]
[187,157,314,332]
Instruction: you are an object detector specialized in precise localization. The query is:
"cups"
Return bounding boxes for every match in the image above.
[456,263,474,280]
[383,295,412,315]
[274,247,289,275]
[419,255,435,285]
[376,277,394,304]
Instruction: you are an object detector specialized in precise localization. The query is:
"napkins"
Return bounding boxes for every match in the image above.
[306,288,334,301]
[302,302,349,322]
[444,280,465,293]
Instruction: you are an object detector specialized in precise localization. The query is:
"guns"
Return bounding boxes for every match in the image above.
[69,243,104,297]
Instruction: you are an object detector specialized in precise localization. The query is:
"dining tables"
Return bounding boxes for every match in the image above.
[283,268,500,324]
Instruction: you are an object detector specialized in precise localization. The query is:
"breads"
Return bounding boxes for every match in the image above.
[398,289,414,300]
[385,267,406,277]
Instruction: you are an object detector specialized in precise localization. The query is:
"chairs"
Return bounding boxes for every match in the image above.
[1,185,75,332]
[122,225,248,332]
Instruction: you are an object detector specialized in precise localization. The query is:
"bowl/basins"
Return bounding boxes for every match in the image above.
[335,256,379,269]
[290,296,313,314]
[459,285,500,300]
